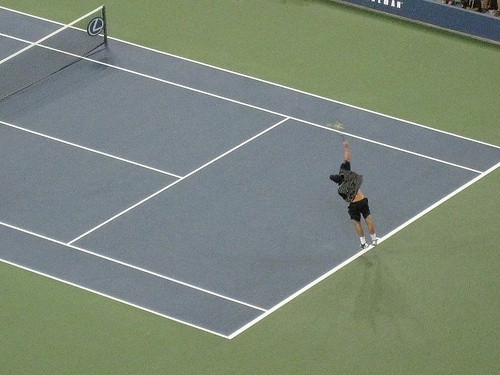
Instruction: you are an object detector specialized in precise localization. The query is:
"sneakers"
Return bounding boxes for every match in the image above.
[360,243,369,249]
[372,239,379,245]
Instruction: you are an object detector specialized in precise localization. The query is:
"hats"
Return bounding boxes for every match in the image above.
[330,175,341,182]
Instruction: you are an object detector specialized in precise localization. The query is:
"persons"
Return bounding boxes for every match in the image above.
[330,140,377,249]
[441,0,500,17]
